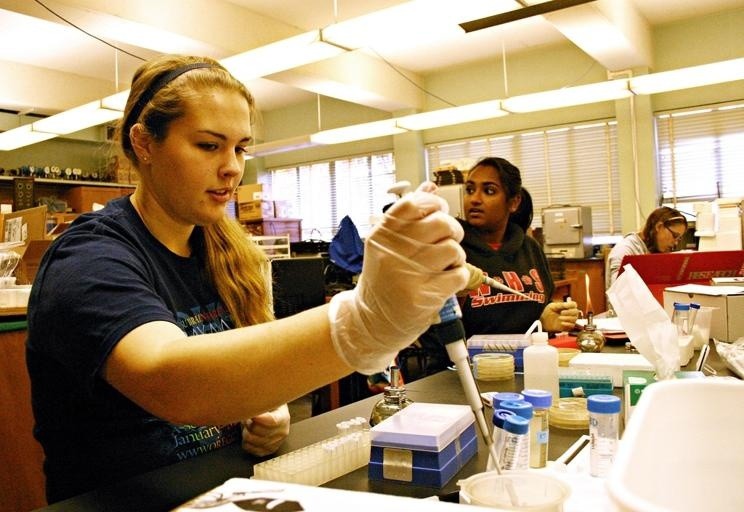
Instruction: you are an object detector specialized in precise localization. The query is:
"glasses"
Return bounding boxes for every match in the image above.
[665,225,683,241]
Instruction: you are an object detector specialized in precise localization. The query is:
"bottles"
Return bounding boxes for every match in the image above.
[522,317,562,399]
[576,311,607,353]
[671,300,701,336]
[587,391,622,479]
[485,387,553,472]
[368,364,418,430]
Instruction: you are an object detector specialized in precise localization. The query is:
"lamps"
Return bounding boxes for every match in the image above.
[0,2,744,158]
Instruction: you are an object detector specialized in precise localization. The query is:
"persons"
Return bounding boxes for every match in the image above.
[25,55,470,506]
[419,157,580,373]
[605,206,688,291]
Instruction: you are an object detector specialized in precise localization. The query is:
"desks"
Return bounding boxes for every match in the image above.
[27,328,707,511]
[548,254,606,315]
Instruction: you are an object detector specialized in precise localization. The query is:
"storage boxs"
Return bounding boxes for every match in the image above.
[2,201,51,289]
[237,182,304,244]
[69,185,125,220]
